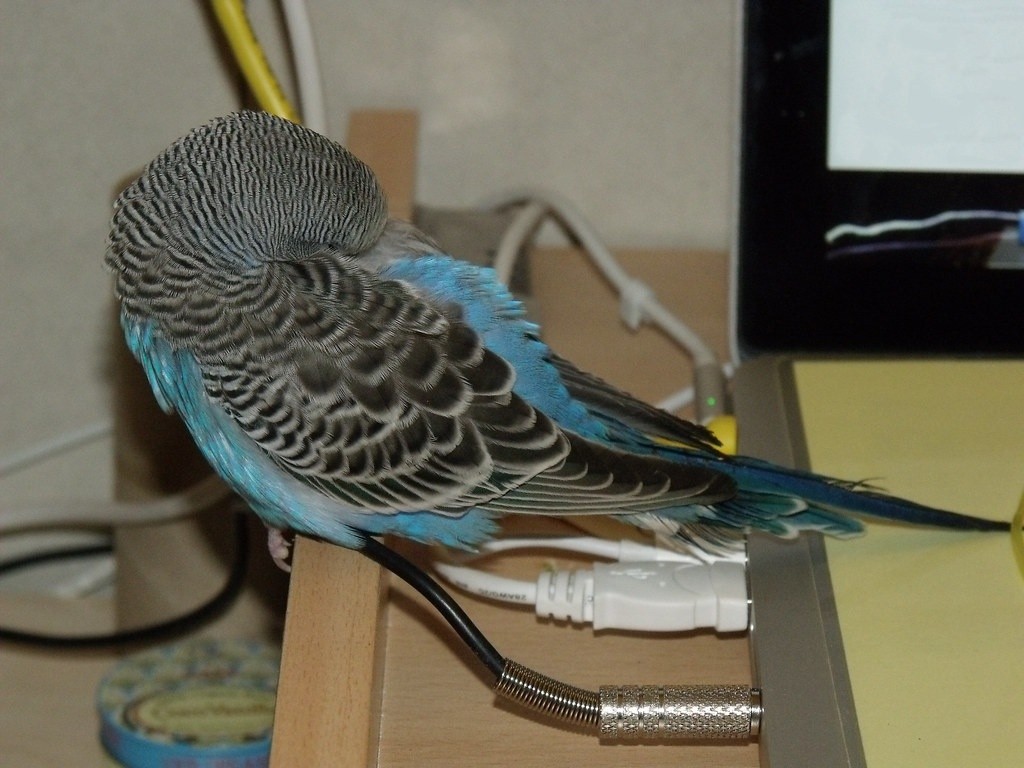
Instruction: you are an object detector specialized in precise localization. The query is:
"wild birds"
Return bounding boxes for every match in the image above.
[104,111,1023,566]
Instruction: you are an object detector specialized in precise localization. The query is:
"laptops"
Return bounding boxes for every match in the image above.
[724,0,1024,768]
